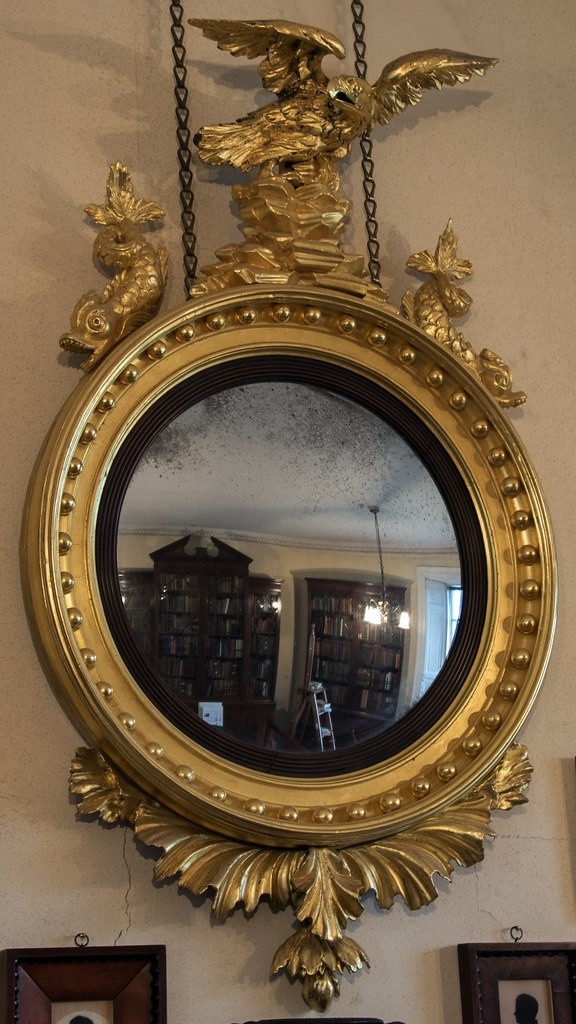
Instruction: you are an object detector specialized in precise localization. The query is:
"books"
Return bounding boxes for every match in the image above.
[122,573,277,697]
[312,596,404,716]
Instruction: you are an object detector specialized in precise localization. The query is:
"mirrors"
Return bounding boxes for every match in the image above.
[18,17,559,1015]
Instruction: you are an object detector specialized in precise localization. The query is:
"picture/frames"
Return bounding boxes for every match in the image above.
[456,943,575,1024]
[1,945,167,1024]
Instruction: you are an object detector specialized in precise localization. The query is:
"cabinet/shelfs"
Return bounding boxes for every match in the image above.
[115,533,404,750]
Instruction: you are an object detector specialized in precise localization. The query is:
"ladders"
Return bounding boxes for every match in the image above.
[287,690,338,752]
